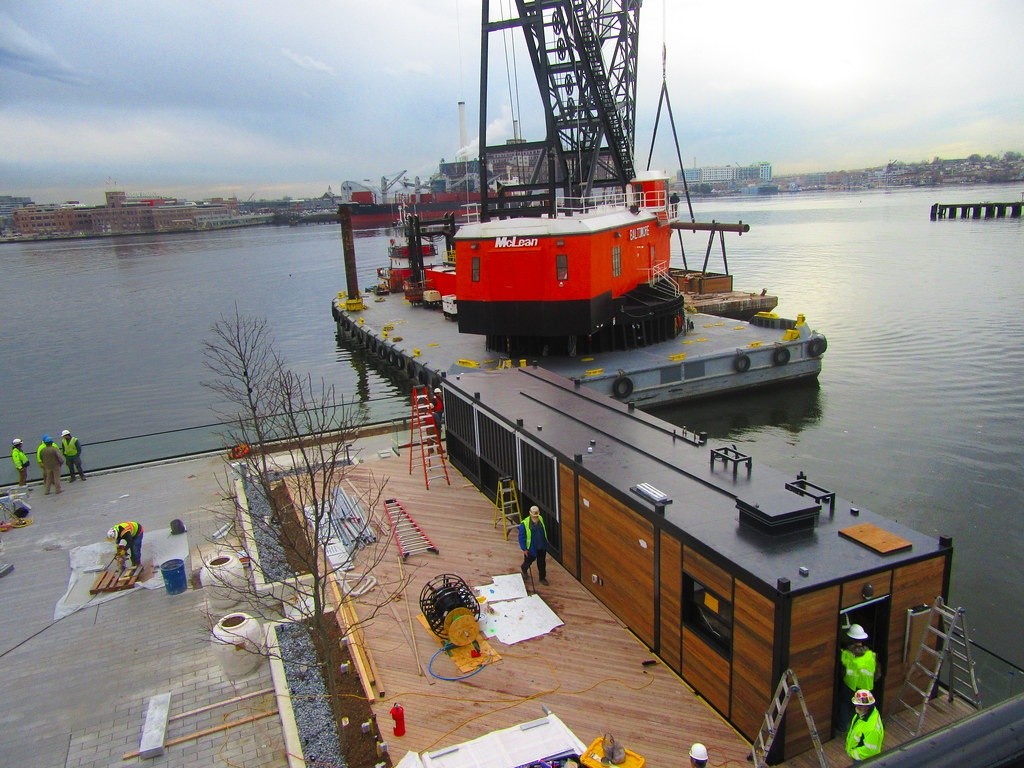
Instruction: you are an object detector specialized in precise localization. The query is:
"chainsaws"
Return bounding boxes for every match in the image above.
[115,545,129,573]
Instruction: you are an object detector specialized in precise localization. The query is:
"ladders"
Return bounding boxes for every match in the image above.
[883,596,982,740]
[748,670,833,768]
[494,476,522,541]
[383,498,439,561]
[409,385,451,490]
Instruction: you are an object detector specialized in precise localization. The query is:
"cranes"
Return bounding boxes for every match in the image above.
[451,0,684,358]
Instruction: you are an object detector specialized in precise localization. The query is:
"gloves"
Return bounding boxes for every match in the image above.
[118,550,126,556]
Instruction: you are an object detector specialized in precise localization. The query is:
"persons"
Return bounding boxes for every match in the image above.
[426,403,446,454]
[108,521,144,566]
[518,506,550,585]
[670,193,680,214]
[429,388,444,432]
[61,430,86,483]
[845,688,884,765]
[835,623,881,735]
[36,434,64,495]
[688,743,708,768]
[10,438,30,486]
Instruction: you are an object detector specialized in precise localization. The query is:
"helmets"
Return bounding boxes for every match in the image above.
[846,624,869,640]
[529,506,540,516]
[426,403,435,410]
[689,742,708,760]
[13,438,23,445]
[851,690,875,705]
[107,529,116,539]
[42,434,49,441]
[434,388,442,393]
[45,437,54,443]
[61,430,70,437]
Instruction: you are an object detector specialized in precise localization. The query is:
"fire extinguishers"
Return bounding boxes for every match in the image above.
[389,703,405,736]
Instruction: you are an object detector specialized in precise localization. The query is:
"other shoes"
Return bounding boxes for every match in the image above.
[437,449,446,454]
[539,578,549,586]
[521,564,529,578]
[131,566,144,572]
[430,449,434,453]
[81,475,87,481]
[69,477,76,483]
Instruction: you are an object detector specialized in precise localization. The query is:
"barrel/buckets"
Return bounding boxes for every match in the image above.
[160,559,187,595]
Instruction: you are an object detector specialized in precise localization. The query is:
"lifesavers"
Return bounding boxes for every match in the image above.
[419,370,427,386]
[809,334,827,357]
[363,335,369,348]
[350,325,357,340]
[229,445,250,459]
[371,337,376,352]
[386,351,395,366]
[734,355,750,371]
[613,377,633,398]
[343,320,349,331]
[432,375,439,390]
[378,345,386,359]
[407,362,414,378]
[337,314,343,325]
[332,307,338,320]
[394,355,404,370]
[774,348,790,366]
[357,330,362,344]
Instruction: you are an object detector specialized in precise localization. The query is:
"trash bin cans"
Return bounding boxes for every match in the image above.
[160,559,188,596]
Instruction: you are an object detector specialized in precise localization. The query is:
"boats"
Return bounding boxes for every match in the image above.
[337,169,513,229]
